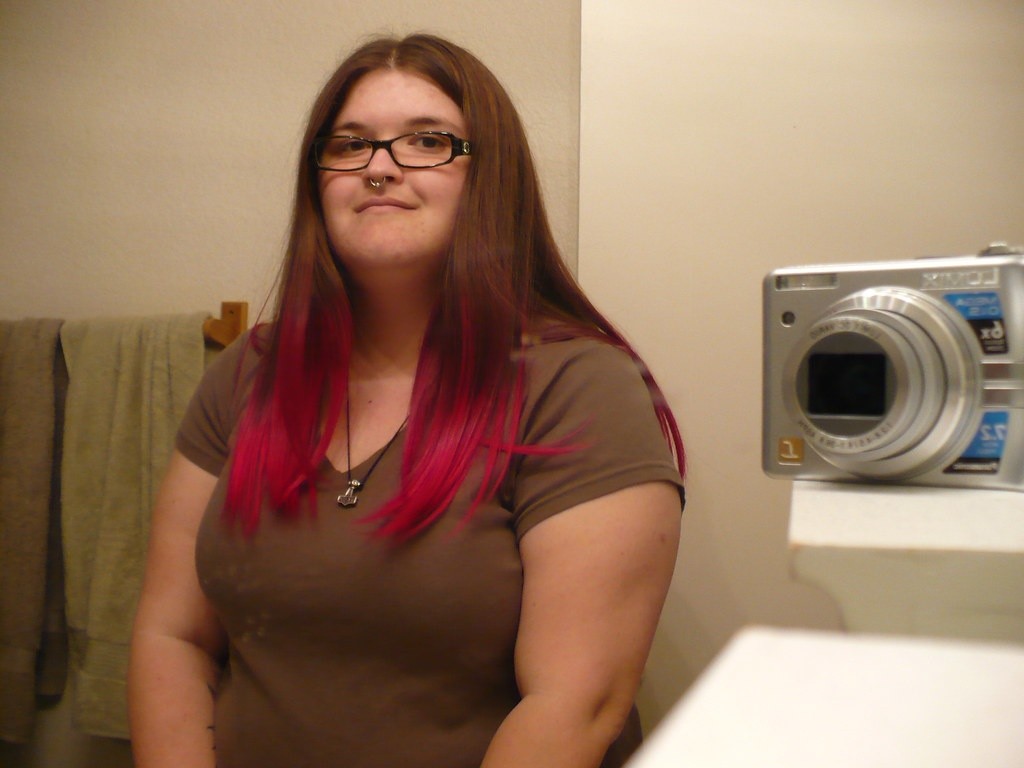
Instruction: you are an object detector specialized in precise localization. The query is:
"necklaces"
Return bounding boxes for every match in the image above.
[336,371,409,507]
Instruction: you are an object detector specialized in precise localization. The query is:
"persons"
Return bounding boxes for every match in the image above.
[127,34,687,768]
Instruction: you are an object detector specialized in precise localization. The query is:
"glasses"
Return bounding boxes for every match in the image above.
[313,131,472,172]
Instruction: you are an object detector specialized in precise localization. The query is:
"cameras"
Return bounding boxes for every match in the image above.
[763,243,1024,495]
